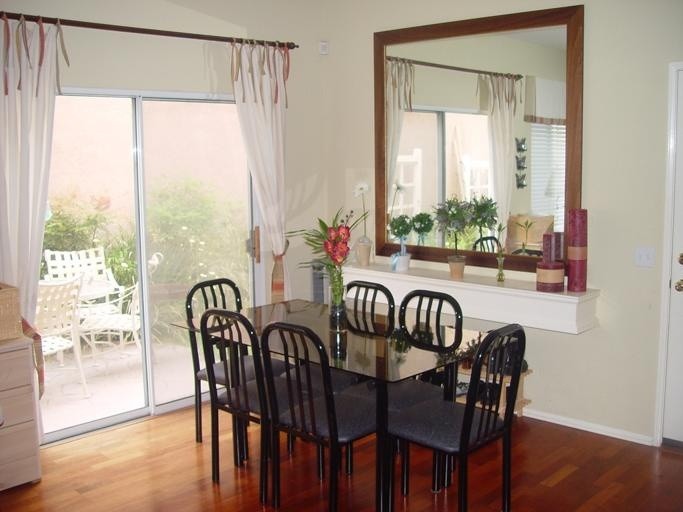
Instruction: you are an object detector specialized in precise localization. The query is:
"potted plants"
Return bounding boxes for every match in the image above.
[388,191,538,282]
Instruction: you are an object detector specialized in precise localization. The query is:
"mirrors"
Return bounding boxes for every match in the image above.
[371,4,583,276]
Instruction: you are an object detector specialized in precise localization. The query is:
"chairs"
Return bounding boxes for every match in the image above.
[34,247,141,401]
[199,308,325,506]
[281,279,396,394]
[261,322,376,512]
[343,290,464,472]
[388,323,525,511]
[185,278,293,460]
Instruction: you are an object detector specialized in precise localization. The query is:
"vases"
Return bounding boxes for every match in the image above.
[280,208,374,300]
[326,283,350,332]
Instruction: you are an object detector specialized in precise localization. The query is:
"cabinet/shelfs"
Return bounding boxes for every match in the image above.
[0,334,41,490]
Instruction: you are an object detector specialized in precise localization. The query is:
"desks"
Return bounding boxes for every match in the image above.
[169,298,520,512]
